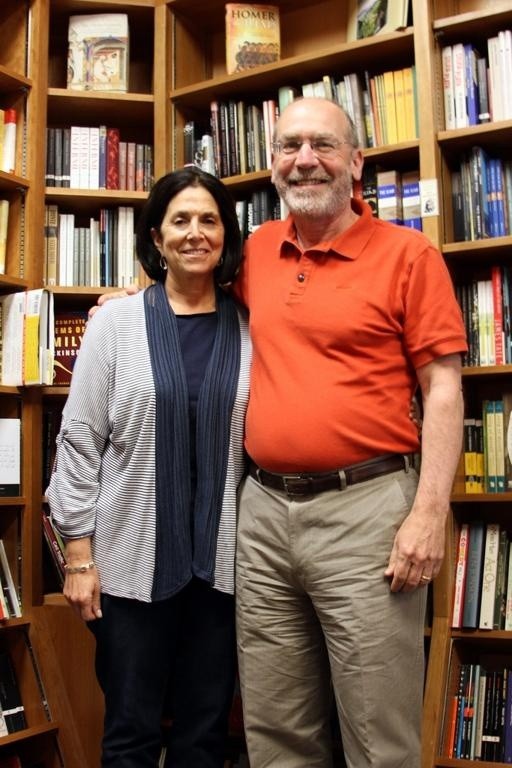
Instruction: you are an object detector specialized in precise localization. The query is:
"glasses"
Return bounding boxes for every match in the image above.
[269,139,348,157]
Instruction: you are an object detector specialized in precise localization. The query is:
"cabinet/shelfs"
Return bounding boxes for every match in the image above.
[0,0,512,768]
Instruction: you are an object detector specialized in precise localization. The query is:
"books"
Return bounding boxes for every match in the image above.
[183,0,422,242]
[441,30,512,764]
[1,15,155,768]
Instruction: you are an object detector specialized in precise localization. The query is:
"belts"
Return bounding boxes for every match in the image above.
[244,455,407,500]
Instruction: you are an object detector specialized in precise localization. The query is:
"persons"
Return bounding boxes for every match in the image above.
[45,166,422,768]
[85,97,469,768]
